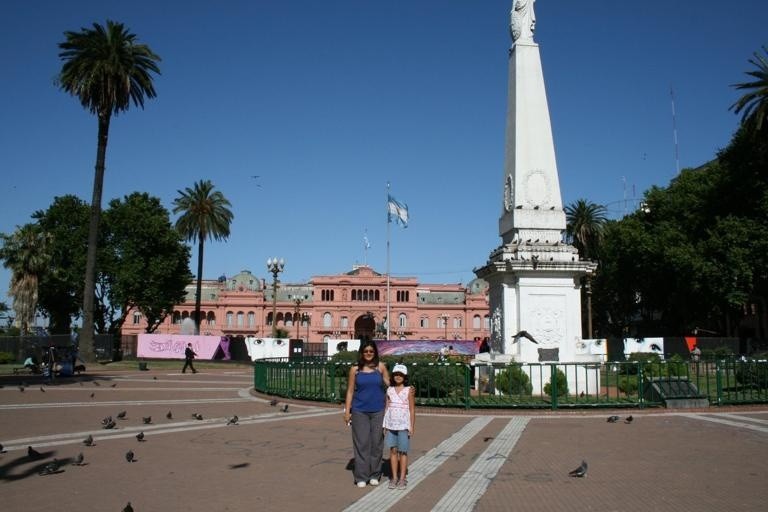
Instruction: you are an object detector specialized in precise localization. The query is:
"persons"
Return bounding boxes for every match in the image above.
[441,337,489,361]
[344,340,417,488]
[382,365,415,489]
[220,336,242,360]
[624,337,664,362]
[182,343,198,373]
[24,357,37,370]
[691,345,701,370]
[246,338,289,363]
[577,340,607,363]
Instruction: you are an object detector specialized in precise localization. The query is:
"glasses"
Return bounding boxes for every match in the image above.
[363,351,373,353]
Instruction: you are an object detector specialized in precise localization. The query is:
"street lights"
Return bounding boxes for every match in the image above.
[288,293,305,339]
[579,256,599,339]
[266,257,285,337]
[441,311,450,339]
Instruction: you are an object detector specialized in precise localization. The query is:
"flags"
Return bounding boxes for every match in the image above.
[387,196,409,229]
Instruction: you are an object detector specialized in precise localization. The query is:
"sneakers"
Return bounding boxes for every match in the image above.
[369,479,378,486]
[357,481,366,488]
[396,479,408,490]
[387,479,398,490]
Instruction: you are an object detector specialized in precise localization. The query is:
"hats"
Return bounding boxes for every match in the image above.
[391,365,407,375]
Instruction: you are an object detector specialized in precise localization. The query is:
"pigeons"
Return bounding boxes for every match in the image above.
[511,330,539,346]
[79,380,118,399]
[607,415,620,423]
[626,415,634,422]
[568,460,587,478]
[122,501,137,512]
[269,399,290,412]
[27,408,241,475]
[1,381,46,394]
